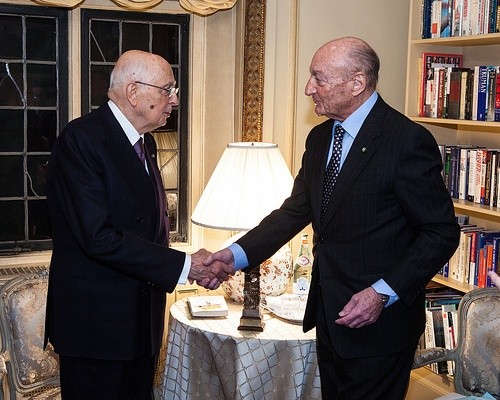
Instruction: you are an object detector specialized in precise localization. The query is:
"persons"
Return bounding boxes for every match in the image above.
[42,49,236,400]
[187,35,462,400]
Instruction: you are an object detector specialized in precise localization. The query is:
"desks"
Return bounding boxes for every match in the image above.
[157,296,321,399]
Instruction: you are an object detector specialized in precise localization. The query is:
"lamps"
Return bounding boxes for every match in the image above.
[191,141,295,332]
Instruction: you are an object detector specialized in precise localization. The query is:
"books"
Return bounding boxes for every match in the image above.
[437,145,500,210]
[416,52,500,124]
[186,295,230,317]
[419,1,500,40]
[435,215,500,289]
[418,283,465,379]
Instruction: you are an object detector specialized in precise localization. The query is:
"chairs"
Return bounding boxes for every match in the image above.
[411,288,500,399]
[0,271,61,400]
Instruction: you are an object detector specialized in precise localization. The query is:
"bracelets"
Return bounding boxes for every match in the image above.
[381,293,390,305]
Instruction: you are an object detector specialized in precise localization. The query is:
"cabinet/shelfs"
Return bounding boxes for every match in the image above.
[405,0,500,379]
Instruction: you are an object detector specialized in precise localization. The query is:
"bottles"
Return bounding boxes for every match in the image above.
[294,234,314,295]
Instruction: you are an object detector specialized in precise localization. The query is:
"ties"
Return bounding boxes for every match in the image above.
[321,125,346,217]
[134,137,143,160]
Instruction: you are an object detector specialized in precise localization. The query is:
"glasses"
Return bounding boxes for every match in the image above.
[135,80,180,97]
